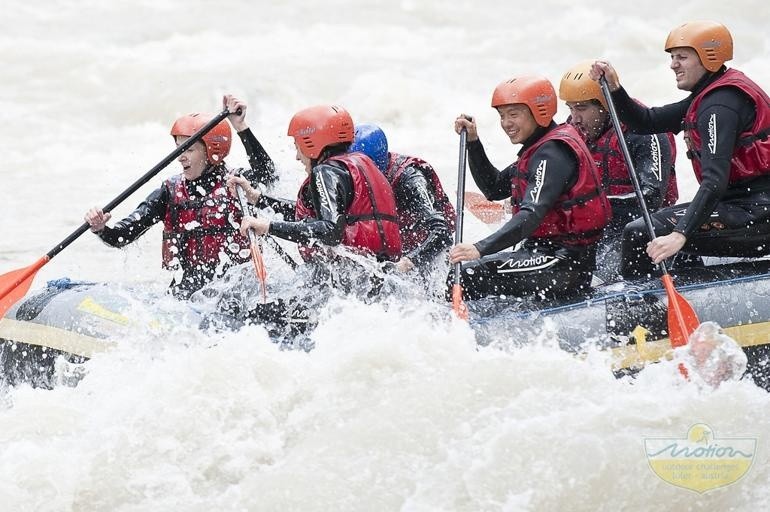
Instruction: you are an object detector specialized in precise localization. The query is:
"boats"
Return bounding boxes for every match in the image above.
[0,273,766,395]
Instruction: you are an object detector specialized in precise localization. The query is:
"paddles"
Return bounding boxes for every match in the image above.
[229,167,270,308]
[448,116,470,324]
[1,97,244,320]
[591,69,701,383]
[455,191,509,224]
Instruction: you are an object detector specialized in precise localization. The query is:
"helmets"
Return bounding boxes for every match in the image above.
[287,105,353,159]
[559,61,621,115]
[666,20,733,72]
[172,113,232,164]
[348,123,389,174]
[492,76,557,126]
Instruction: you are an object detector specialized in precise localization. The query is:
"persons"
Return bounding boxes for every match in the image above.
[227,106,402,304]
[84,94,280,299]
[445,77,612,303]
[589,20,770,285]
[347,125,457,277]
[559,63,679,281]
